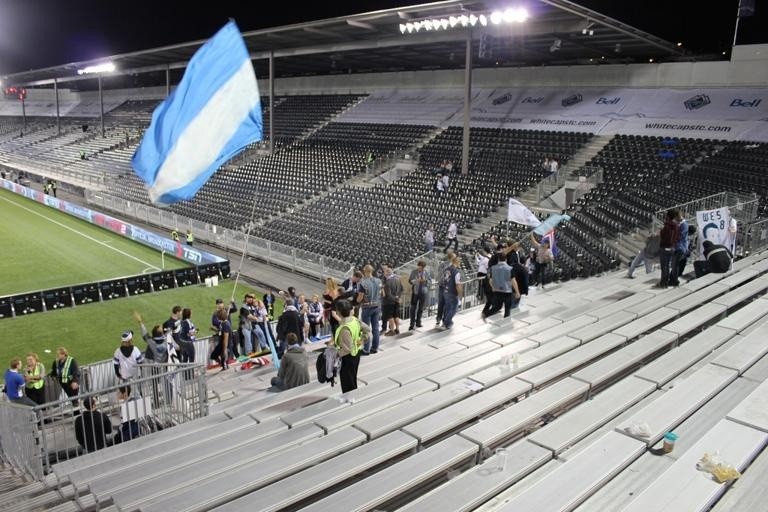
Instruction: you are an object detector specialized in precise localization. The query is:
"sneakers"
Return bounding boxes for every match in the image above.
[395,330,399,334]
[385,330,395,336]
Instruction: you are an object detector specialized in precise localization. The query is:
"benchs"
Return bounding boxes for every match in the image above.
[3,246,768,512]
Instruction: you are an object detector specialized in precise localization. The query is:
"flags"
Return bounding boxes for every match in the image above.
[131,19,264,210]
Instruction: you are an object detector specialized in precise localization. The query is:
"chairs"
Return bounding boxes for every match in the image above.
[83,87,445,247]
[298,125,768,299]
[2,112,102,196]
